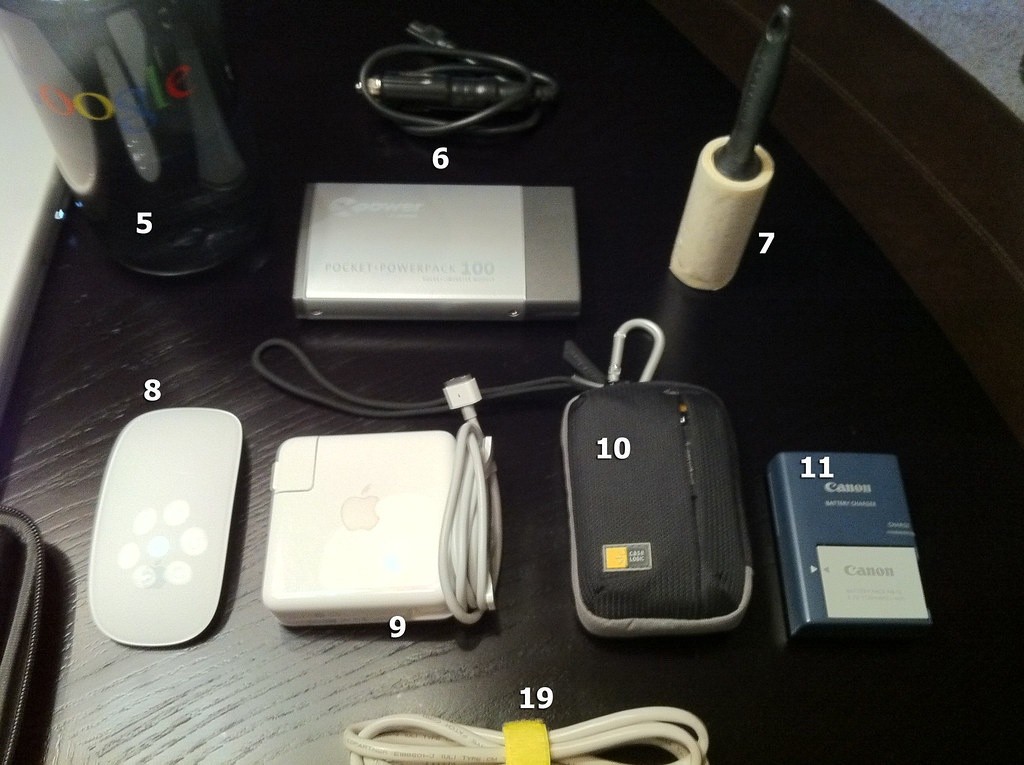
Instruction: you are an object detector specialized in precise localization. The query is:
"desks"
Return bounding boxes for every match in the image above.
[0,1,1023,764]
